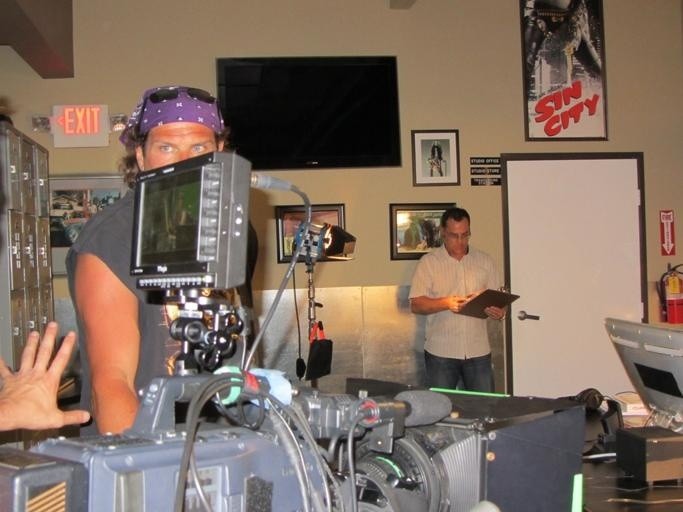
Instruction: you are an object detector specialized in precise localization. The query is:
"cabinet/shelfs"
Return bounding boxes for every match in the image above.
[1,122,54,369]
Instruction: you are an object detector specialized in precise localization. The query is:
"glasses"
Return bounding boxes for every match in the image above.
[440,228,471,241]
[145,85,217,104]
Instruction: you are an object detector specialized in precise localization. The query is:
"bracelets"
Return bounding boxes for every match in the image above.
[499,312,506,321]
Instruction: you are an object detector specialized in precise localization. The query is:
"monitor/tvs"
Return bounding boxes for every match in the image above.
[601,317,683,433]
[215,55,401,170]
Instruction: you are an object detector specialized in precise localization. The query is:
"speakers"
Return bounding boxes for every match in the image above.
[616,427,683,487]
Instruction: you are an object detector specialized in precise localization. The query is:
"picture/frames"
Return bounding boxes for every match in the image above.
[520,1,609,141]
[48,173,129,277]
[410,131,460,187]
[389,203,456,260]
[274,204,346,262]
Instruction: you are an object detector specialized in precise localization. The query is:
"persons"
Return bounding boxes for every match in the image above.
[431,140,444,176]
[67,84,257,447]
[408,209,506,394]
[0,321,89,432]
